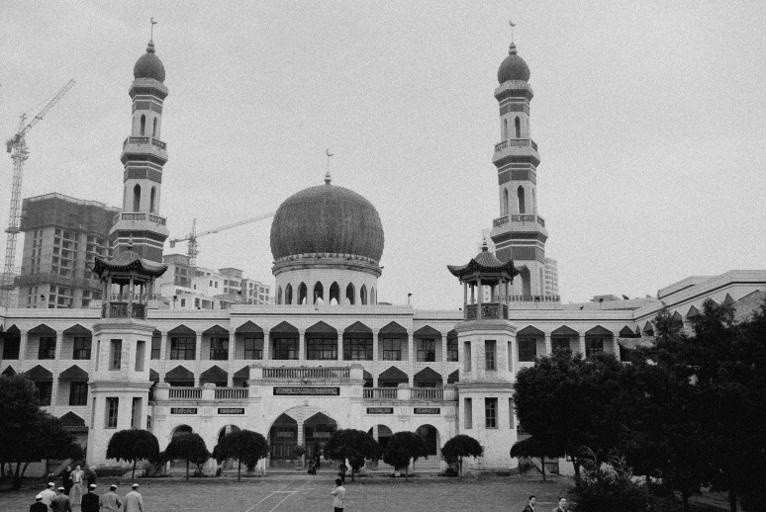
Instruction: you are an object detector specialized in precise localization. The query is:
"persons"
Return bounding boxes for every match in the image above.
[330,479,345,512]
[306,448,321,476]
[552,497,568,512]
[29,465,143,512]
[337,463,348,484]
[523,495,536,512]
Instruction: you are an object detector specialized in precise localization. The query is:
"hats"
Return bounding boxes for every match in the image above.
[36,481,140,500]
